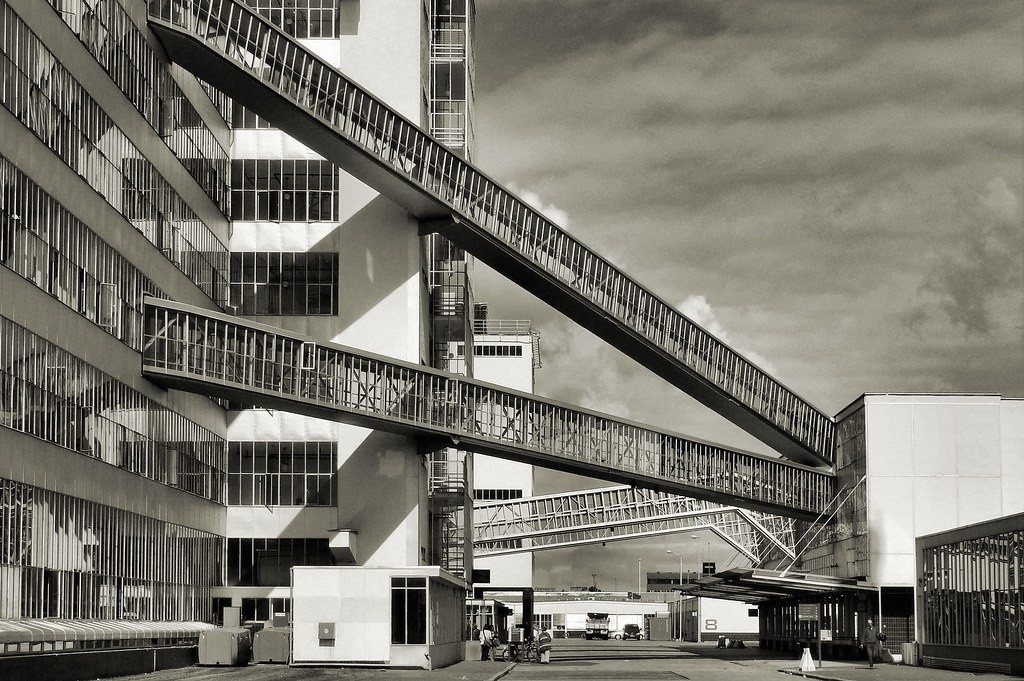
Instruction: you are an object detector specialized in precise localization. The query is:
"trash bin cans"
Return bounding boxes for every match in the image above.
[900,641,919,665]
[197,627,250,667]
[253,626,290,663]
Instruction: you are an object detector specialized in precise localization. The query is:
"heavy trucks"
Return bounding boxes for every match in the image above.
[565,612,649,640]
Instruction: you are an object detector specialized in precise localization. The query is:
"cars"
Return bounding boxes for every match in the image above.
[610,631,624,640]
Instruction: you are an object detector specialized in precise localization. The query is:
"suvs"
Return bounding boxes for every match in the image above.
[623,624,642,641]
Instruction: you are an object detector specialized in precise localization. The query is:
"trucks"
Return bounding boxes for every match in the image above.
[585,612,610,640]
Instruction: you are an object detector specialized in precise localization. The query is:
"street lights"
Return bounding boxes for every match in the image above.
[690,535,710,577]
[666,549,682,586]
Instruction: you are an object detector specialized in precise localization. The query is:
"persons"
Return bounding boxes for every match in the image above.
[479,624,492,662]
[859,619,886,670]
[536,626,551,663]
[490,625,500,662]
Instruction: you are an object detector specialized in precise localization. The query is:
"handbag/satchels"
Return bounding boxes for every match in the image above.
[484,639,492,647]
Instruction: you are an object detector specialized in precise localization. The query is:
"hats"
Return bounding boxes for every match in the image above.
[541,626,547,631]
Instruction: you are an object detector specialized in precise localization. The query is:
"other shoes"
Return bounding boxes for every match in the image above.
[481,660,489,663]
[869,664,873,670]
[541,662,550,664]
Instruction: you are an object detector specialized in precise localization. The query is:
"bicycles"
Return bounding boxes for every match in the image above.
[501,636,539,664]
